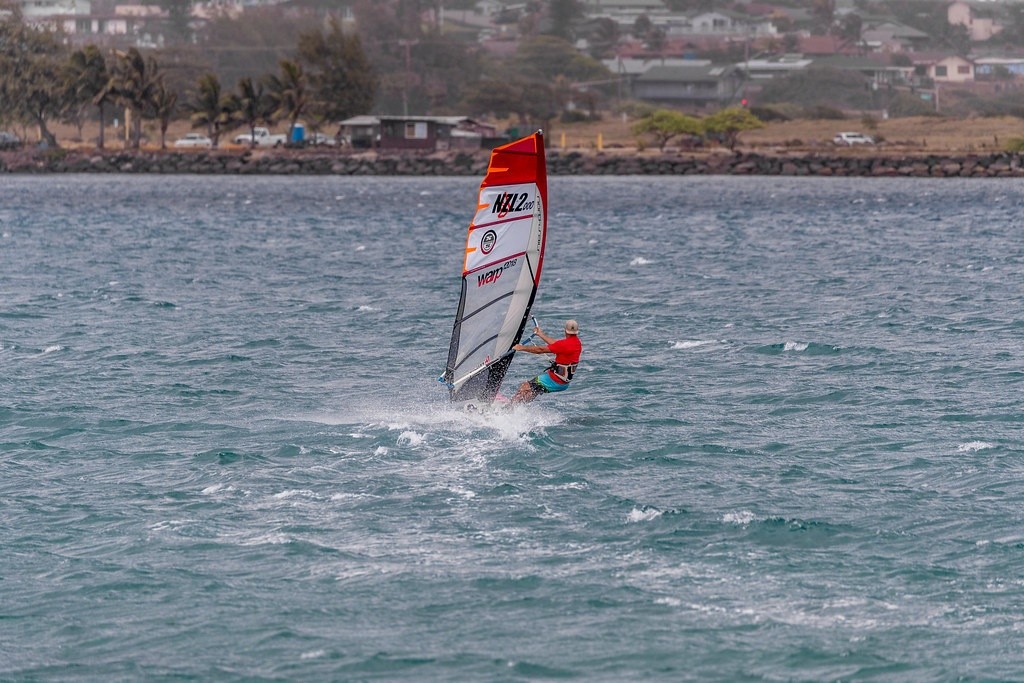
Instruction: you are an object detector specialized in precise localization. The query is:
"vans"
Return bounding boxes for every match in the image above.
[834,131,874,144]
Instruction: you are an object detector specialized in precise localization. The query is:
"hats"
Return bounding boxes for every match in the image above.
[565,320,578,334]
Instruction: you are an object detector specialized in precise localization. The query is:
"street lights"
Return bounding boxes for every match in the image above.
[724,36,751,88]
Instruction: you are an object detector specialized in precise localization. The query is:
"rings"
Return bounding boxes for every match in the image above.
[513,347,514,348]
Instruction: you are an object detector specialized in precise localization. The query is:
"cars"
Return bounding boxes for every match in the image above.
[0,132,21,150]
[173,133,212,149]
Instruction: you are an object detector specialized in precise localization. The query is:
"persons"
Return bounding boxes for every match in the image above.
[511,319,582,403]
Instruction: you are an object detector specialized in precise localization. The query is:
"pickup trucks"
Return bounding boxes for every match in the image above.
[235,127,287,149]
